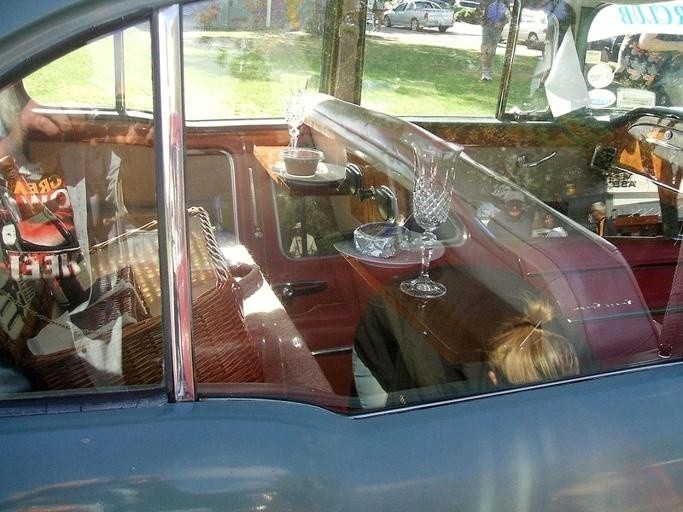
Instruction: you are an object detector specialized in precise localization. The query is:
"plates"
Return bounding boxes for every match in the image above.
[581,63,617,112]
[332,227,444,268]
[270,159,352,188]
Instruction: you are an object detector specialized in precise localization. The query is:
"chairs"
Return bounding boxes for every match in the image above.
[300,86,661,367]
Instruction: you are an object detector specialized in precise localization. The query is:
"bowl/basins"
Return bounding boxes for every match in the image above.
[280,149,322,177]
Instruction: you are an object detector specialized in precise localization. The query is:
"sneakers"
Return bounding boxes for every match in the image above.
[481,73,492,80]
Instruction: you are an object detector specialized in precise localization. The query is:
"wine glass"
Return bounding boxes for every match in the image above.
[401,141,464,299]
[281,94,308,152]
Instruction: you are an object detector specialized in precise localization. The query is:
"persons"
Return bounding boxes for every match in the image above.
[486,191,533,240]
[613,34,683,91]
[372,0,386,32]
[290,222,318,258]
[481,0,510,80]
[353,265,580,409]
[532,212,568,238]
[0,79,155,168]
[591,202,617,235]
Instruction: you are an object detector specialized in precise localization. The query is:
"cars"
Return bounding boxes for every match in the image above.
[500,6,554,44]
[382,0,480,31]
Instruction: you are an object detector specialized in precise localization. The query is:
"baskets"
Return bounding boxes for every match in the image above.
[20,207,263,383]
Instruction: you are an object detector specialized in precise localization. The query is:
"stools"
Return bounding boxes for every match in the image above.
[147,226,332,396]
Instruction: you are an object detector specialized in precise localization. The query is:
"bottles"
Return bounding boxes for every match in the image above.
[0,155,96,311]
